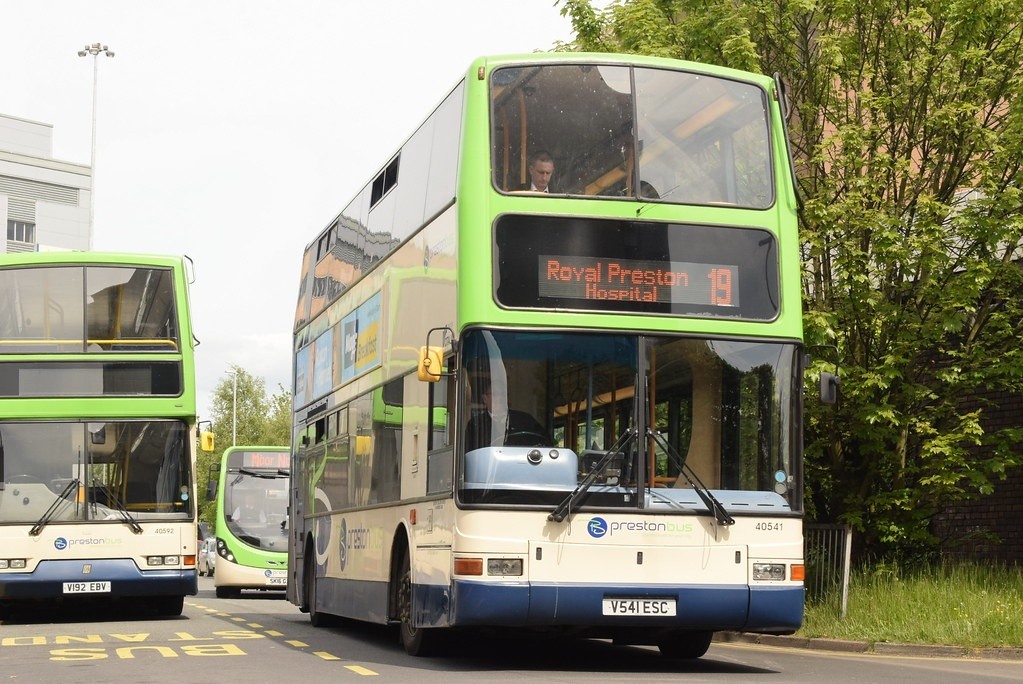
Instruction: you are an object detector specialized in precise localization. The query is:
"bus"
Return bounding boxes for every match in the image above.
[286,53,844,658]
[365,266,458,429]
[1,251,216,620]
[310,436,377,516]
[203,446,292,595]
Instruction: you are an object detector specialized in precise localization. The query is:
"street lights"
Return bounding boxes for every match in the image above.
[77,42,116,250]
[225,370,237,446]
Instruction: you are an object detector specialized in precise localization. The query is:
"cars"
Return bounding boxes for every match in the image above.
[199,539,217,577]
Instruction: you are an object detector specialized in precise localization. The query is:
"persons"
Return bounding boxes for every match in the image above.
[231,495,266,523]
[465,379,551,452]
[511,150,557,194]
